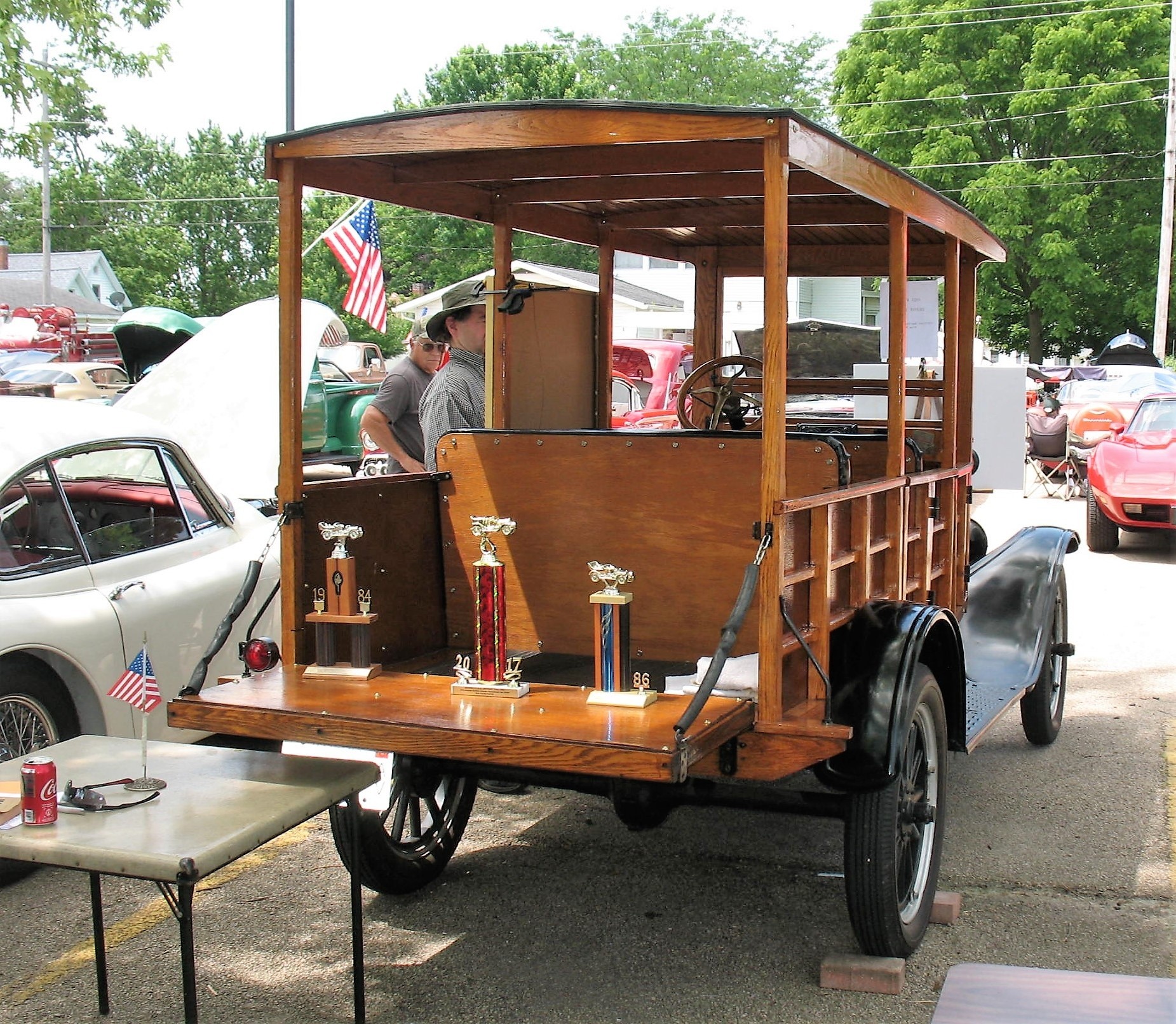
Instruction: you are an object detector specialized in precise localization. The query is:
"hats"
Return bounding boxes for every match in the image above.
[426,279,486,344]
[412,314,435,339]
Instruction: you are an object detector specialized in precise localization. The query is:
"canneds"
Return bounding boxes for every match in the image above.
[20,757,58,827]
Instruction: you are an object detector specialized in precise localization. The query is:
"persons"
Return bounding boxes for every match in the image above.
[359,314,450,474]
[1042,397,1110,498]
[418,279,488,470]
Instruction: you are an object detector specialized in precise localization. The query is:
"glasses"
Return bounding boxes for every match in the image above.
[417,341,444,352]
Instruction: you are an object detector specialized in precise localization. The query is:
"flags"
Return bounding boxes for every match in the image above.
[322,198,388,335]
[106,649,162,714]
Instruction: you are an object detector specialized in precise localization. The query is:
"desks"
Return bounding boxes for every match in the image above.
[0,734,385,1024]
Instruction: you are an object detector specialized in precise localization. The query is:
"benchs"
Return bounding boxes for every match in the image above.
[434,426,924,671]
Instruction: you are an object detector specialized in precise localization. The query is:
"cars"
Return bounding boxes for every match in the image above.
[0,288,881,895]
[1082,392,1176,553]
[1025,367,1166,485]
[166,95,1084,966]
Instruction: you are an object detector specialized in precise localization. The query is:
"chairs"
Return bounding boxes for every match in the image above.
[1026,413,1087,500]
[1153,411,1176,429]
